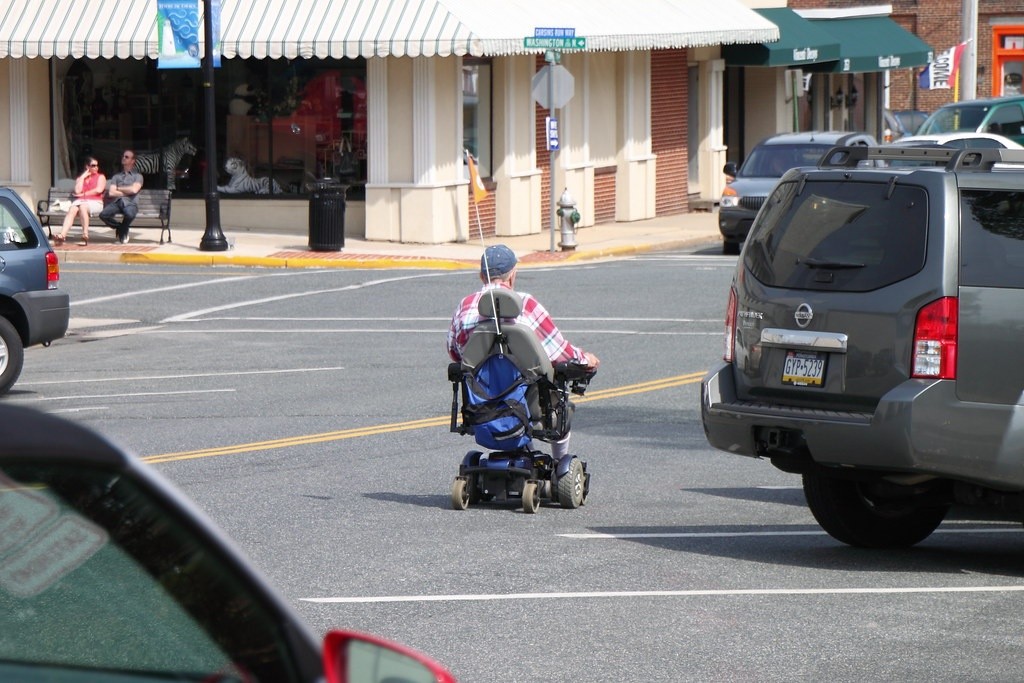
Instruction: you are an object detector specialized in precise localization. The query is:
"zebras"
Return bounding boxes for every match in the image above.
[132,137,197,189]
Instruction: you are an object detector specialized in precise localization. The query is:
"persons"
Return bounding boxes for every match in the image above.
[99,149,144,245]
[52,154,106,245]
[447,245,599,462]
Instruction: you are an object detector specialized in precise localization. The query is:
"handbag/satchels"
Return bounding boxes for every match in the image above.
[465,355,531,450]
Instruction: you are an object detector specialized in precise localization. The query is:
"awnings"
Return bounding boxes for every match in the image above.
[0,0,779,62]
[720,7,935,75]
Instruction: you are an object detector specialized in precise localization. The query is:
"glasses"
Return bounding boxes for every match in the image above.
[122,155,129,159]
[90,164,99,168]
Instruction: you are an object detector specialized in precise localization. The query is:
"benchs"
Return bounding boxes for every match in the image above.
[37,187,172,245]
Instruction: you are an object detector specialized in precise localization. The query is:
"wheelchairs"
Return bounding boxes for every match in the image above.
[445,287,597,513]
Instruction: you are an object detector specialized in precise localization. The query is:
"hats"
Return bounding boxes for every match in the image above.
[480,244,519,276]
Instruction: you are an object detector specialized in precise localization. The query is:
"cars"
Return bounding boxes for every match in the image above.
[0,185,73,397]
[874,96,1024,170]
[716,130,884,253]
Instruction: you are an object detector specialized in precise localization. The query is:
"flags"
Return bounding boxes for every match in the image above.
[466,154,488,203]
[920,43,967,90]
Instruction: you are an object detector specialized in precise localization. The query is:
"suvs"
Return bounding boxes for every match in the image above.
[697,138,1024,554]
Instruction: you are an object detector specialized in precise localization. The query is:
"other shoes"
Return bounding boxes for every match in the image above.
[54,233,66,243]
[81,235,89,245]
[115,224,129,244]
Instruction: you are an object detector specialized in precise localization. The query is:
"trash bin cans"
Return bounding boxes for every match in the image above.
[305,177,350,251]
